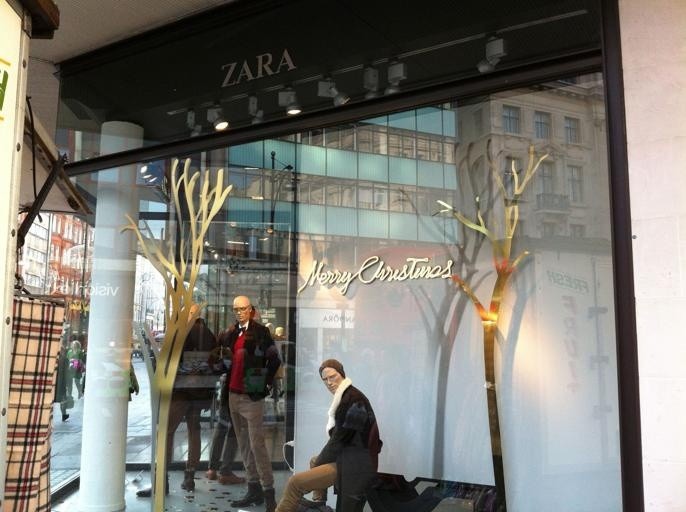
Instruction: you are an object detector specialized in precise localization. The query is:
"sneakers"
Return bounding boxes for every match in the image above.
[62,414,69,420]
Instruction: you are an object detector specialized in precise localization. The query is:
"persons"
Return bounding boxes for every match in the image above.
[204,305,255,485]
[274,358,379,512]
[135,301,218,496]
[52,334,86,421]
[219,295,281,512]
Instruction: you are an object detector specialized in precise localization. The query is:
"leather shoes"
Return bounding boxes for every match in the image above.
[206,469,217,479]
[219,473,245,484]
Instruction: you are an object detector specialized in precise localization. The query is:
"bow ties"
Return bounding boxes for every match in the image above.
[237,327,246,333]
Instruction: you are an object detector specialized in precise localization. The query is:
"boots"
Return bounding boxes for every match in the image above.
[231,482,264,507]
[181,470,196,492]
[136,480,169,496]
[263,488,277,512]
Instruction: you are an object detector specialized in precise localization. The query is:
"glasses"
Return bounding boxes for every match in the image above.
[232,304,250,312]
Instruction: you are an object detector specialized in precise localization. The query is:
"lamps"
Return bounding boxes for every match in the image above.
[477,35,508,74]
[278,86,302,115]
[384,62,410,95]
[248,95,264,125]
[317,79,349,105]
[178,107,203,138]
[361,66,378,99]
[206,104,229,131]
